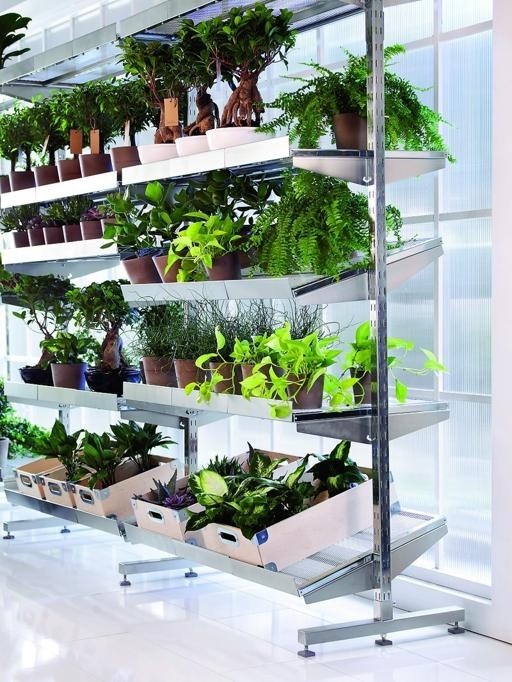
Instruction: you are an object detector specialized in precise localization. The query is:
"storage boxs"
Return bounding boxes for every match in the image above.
[1,399,403,572]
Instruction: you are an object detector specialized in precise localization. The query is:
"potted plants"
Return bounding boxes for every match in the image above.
[2,270,446,420]
[0,169,419,283]
[0,0,459,194]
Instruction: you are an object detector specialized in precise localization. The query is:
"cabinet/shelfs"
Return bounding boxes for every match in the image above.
[0,0,466,657]
